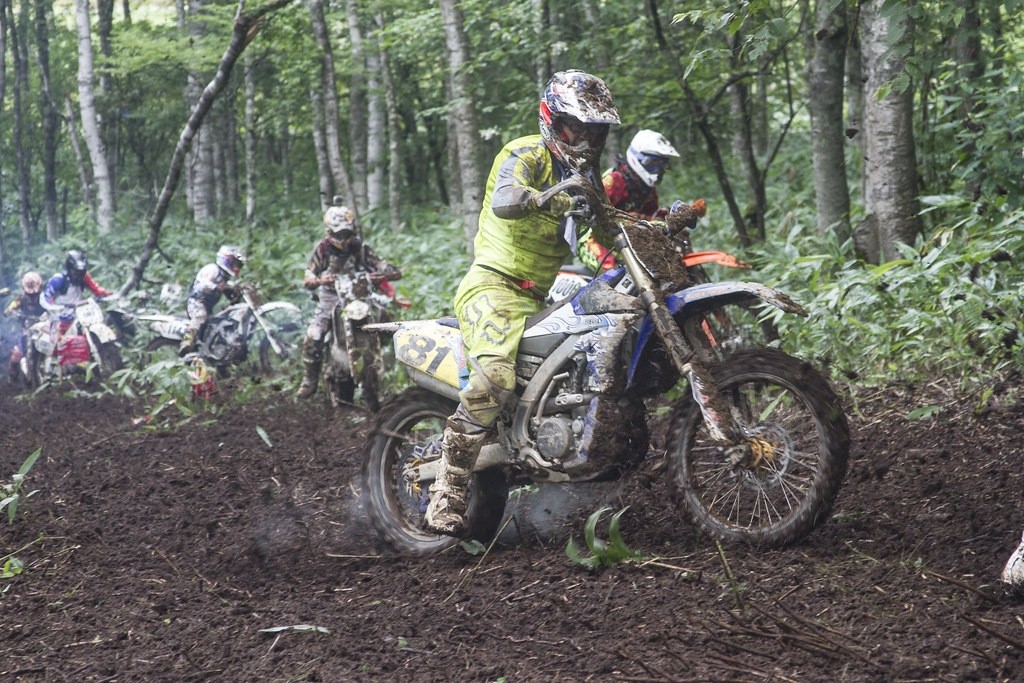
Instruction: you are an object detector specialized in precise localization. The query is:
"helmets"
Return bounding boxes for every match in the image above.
[537,68,622,175]
[62,250,88,286]
[625,129,682,189]
[322,204,357,251]
[21,271,43,295]
[215,245,248,279]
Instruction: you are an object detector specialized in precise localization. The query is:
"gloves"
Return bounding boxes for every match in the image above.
[531,187,577,218]
[645,218,670,235]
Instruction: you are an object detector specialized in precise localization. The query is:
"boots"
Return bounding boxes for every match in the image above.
[177,328,200,358]
[420,414,490,538]
[296,338,325,400]
[7,349,23,386]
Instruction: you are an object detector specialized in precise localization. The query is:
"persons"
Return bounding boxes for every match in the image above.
[574,128,681,280]
[177,243,254,357]
[418,67,697,539]
[5,271,47,387]
[39,249,113,381]
[295,203,401,401]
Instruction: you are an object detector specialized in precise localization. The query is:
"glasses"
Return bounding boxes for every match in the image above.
[328,229,353,239]
[559,118,608,135]
[641,159,672,170]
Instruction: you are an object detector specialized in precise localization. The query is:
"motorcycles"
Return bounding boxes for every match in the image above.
[5,295,124,383]
[321,272,391,412]
[136,282,308,381]
[360,172,852,561]
